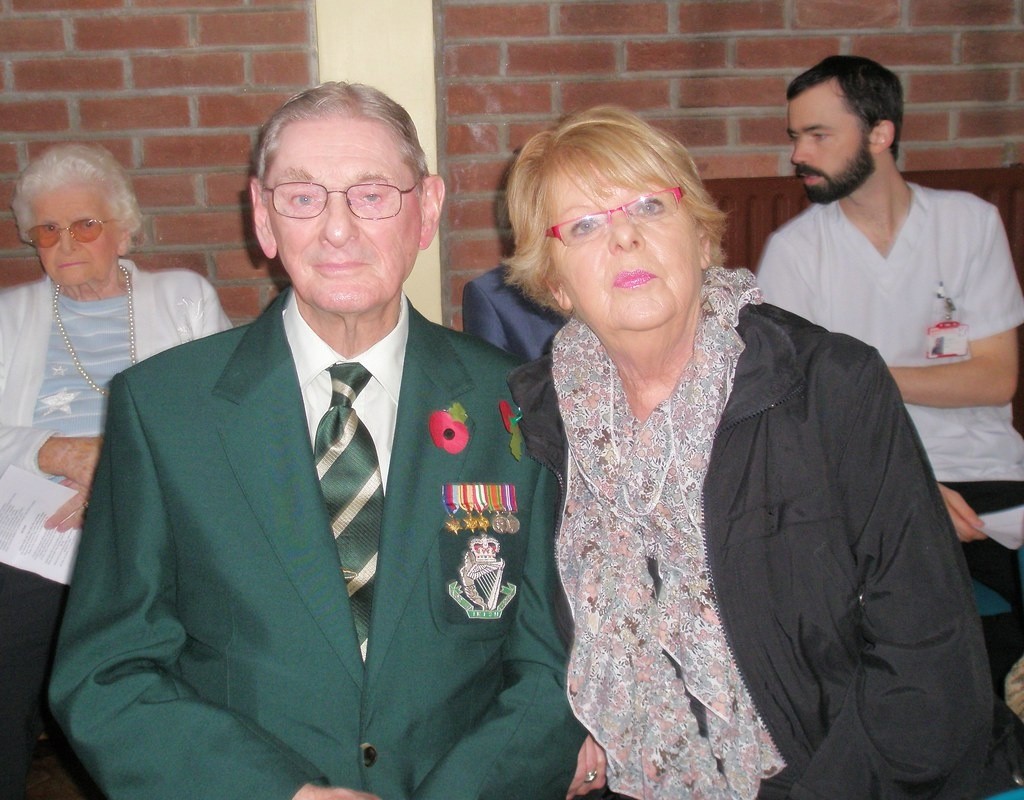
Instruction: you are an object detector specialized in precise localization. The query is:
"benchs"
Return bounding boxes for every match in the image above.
[702,164,1024,623]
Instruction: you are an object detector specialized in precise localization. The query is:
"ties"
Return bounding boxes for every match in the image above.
[313,362,387,667]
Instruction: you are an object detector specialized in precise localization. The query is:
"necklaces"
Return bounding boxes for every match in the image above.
[54,265,135,396]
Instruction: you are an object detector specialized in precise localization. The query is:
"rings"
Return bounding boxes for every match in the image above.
[584,772,596,783]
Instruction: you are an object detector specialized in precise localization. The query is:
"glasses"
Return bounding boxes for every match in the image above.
[542,184,687,247]
[259,174,426,219]
[25,216,121,248]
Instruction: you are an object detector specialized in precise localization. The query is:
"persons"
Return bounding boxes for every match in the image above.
[50,80,590,800]
[498,103,1013,800]
[461,261,568,361]
[931,338,943,354]
[0,140,235,800]
[748,53,1024,800]
